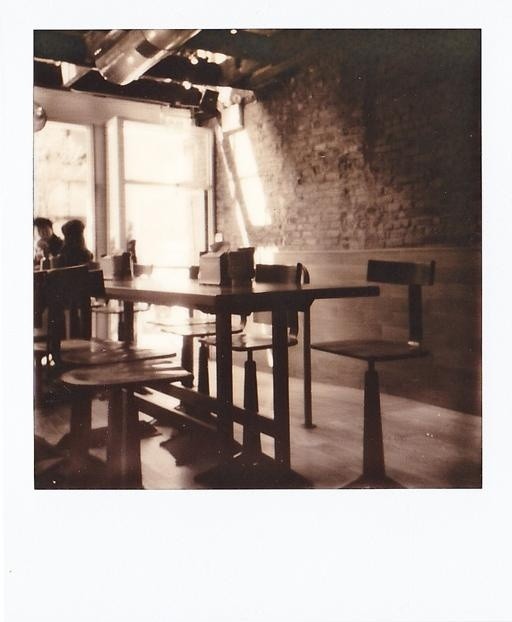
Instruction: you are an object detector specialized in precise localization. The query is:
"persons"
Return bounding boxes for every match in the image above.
[55,220,94,266]
[33,217,63,265]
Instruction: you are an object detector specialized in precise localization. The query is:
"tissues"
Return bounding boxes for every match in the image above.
[100,244,135,282]
